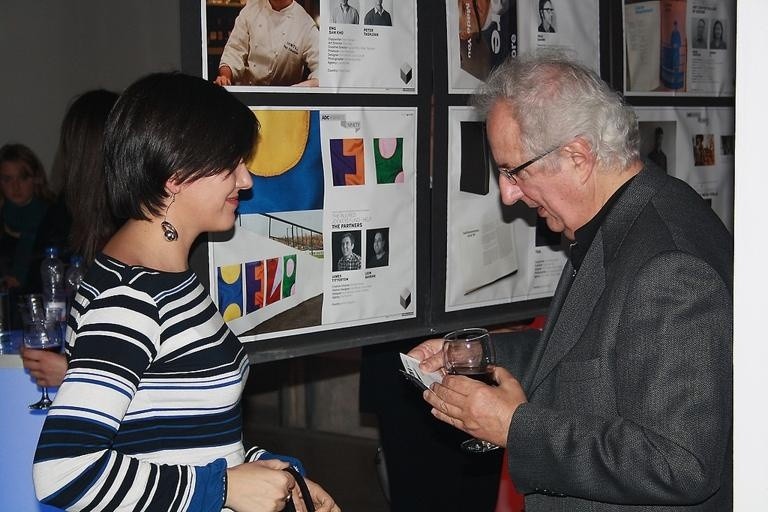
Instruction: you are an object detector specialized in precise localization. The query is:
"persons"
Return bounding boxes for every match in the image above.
[364,0,392,26]
[213,0,319,88]
[367,232,388,267]
[330,0,359,24]
[32,70,342,512]
[710,20,726,49]
[0,143,59,330]
[648,127,667,173]
[694,135,704,166]
[671,21,681,71]
[337,233,361,271]
[538,0,555,33]
[405,44,733,512]
[692,19,707,48]
[20,90,119,386]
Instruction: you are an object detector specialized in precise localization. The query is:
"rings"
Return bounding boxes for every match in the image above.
[287,493,292,500]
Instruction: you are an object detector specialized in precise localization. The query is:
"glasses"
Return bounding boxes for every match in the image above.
[498,135,578,185]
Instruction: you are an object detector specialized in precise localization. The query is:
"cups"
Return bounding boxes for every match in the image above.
[12,294,46,325]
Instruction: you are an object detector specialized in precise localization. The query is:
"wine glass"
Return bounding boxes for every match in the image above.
[23,318,65,411]
[442,328,501,454]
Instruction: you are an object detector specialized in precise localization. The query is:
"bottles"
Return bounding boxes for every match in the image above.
[65,256,87,312]
[0,284,12,354]
[37,246,65,319]
